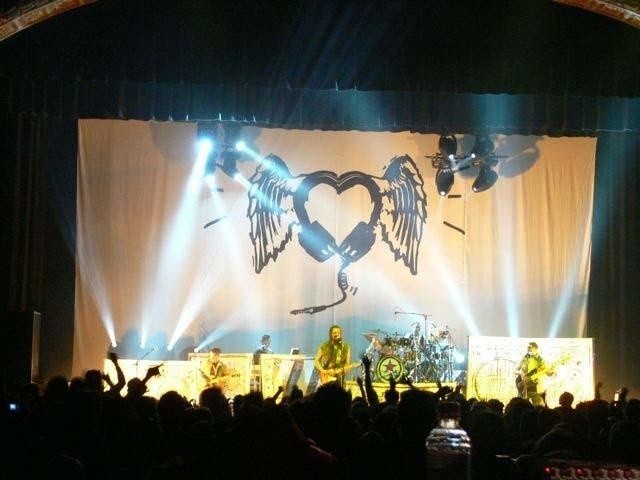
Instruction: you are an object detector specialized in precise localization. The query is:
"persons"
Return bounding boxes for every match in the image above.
[514,341,554,406]
[1,354,639,480]
[313,326,353,392]
[253,334,274,364]
[404,322,427,347]
[199,349,230,395]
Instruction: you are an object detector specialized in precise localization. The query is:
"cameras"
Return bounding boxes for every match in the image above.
[9,403,20,412]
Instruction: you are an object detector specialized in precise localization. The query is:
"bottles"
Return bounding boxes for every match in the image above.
[422,401,479,479]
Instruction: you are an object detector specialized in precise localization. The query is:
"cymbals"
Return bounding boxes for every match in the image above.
[361,329,386,336]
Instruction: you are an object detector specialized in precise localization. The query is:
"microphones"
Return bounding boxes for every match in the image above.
[337,336,342,341]
[393,306,397,320]
[152,344,160,352]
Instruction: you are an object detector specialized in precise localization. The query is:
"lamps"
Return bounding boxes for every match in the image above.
[470,134,494,161]
[471,162,500,192]
[435,134,457,195]
[222,122,245,180]
[196,121,217,176]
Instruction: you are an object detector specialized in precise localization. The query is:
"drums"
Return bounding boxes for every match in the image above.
[382,337,397,346]
[405,350,422,362]
[398,337,412,347]
[376,355,402,383]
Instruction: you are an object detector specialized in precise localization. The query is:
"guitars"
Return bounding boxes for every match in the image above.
[319,361,364,385]
[198,371,241,393]
[516,355,570,393]
